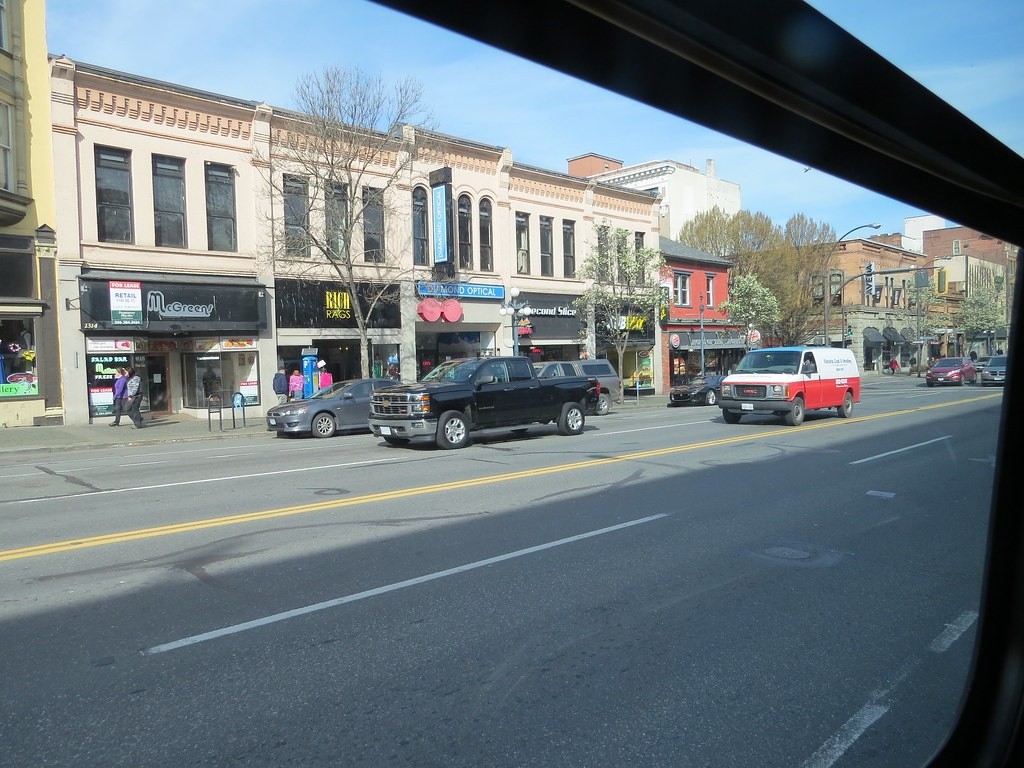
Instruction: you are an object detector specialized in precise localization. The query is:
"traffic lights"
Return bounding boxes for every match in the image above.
[848,326,851,335]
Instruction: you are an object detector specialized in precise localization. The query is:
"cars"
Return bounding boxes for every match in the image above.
[926,357,977,387]
[265,378,404,438]
[669,375,727,405]
[976,356,992,371]
[981,355,1007,386]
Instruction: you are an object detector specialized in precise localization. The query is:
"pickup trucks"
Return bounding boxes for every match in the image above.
[532,359,620,416]
[368,356,601,450]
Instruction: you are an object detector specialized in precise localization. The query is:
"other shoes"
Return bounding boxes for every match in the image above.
[131,424,142,429]
[109,422,119,426]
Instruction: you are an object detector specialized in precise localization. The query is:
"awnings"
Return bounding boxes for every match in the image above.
[883,328,905,346]
[864,328,887,347]
[902,333,924,345]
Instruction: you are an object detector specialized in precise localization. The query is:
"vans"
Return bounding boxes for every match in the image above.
[715,346,861,426]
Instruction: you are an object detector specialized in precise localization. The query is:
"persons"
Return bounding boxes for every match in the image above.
[995,347,1003,356]
[124,368,144,429]
[273,367,288,404]
[374,353,384,379]
[109,368,143,427]
[970,348,977,362]
[910,355,916,367]
[890,356,900,374]
[203,363,216,398]
[289,367,303,399]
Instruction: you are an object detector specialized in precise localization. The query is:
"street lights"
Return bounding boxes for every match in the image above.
[499,287,532,357]
[824,224,881,347]
[983,330,994,356]
[917,257,951,378]
